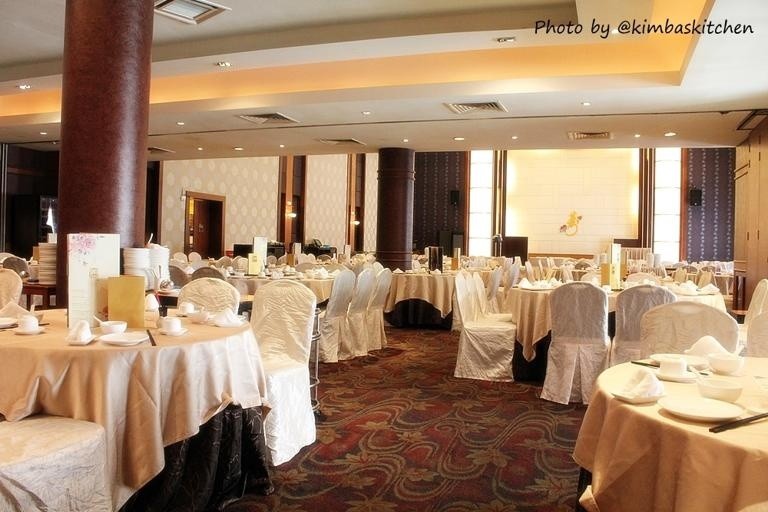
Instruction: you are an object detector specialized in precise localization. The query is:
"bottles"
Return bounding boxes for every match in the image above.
[156,306,168,329]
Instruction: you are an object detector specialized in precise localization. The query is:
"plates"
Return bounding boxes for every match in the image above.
[38,243,57,286]
[391,267,441,277]
[656,394,742,422]
[100,331,150,347]
[213,320,242,328]
[122,246,173,295]
[655,371,694,383]
[0,316,16,329]
[520,276,716,296]
[158,327,188,336]
[649,352,703,369]
[16,327,45,335]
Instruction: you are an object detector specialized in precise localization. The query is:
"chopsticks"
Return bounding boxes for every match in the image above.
[146,329,156,346]
[707,413,767,433]
[630,361,658,369]
[13,323,49,326]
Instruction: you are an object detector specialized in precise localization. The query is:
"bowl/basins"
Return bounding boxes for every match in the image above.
[99,320,127,336]
[188,312,210,324]
[708,352,740,374]
[30,311,43,323]
[694,376,742,404]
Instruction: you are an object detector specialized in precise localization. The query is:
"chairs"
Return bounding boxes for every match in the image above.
[0,252,768,512]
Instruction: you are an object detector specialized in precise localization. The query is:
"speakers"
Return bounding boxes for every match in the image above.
[450,190,460,203]
[690,187,701,206]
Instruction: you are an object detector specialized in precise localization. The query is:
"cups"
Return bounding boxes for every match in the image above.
[218,265,328,281]
[179,303,194,316]
[660,357,688,376]
[163,317,181,333]
[18,314,39,331]
[25,264,38,282]
